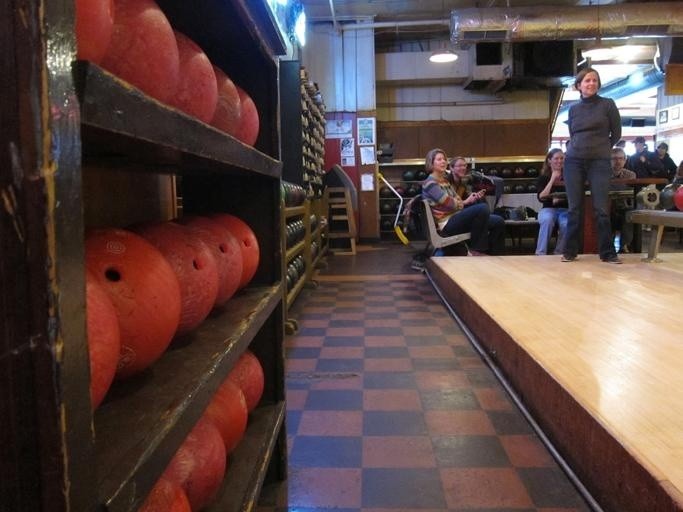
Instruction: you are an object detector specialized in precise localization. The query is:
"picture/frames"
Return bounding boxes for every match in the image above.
[671,107,680,120]
[658,110,668,124]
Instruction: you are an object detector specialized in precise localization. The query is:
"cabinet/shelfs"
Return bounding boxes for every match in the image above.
[278,37,328,336]
[378,155,548,233]
[1,1,289,511]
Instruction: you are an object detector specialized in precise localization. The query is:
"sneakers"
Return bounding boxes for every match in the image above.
[560,252,578,261]
[600,252,622,265]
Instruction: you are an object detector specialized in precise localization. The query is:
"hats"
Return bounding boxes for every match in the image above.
[631,136,645,143]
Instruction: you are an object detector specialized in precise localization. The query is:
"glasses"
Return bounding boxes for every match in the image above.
[454,163,467,168]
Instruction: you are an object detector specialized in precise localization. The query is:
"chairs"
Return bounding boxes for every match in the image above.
[416,199,473,273]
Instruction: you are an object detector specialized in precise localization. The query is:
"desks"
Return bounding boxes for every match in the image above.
[501,218,544,251]
[553,177,668,253]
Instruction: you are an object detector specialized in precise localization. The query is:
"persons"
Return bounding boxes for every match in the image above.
[535,137,683,255]
[446,156,487,255]
[562,67,621,263]
[421,148,506,256]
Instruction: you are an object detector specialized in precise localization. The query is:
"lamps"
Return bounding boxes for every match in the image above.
[429,40,459,63]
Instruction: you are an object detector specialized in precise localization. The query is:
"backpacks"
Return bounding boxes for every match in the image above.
[404,197,427,240]
